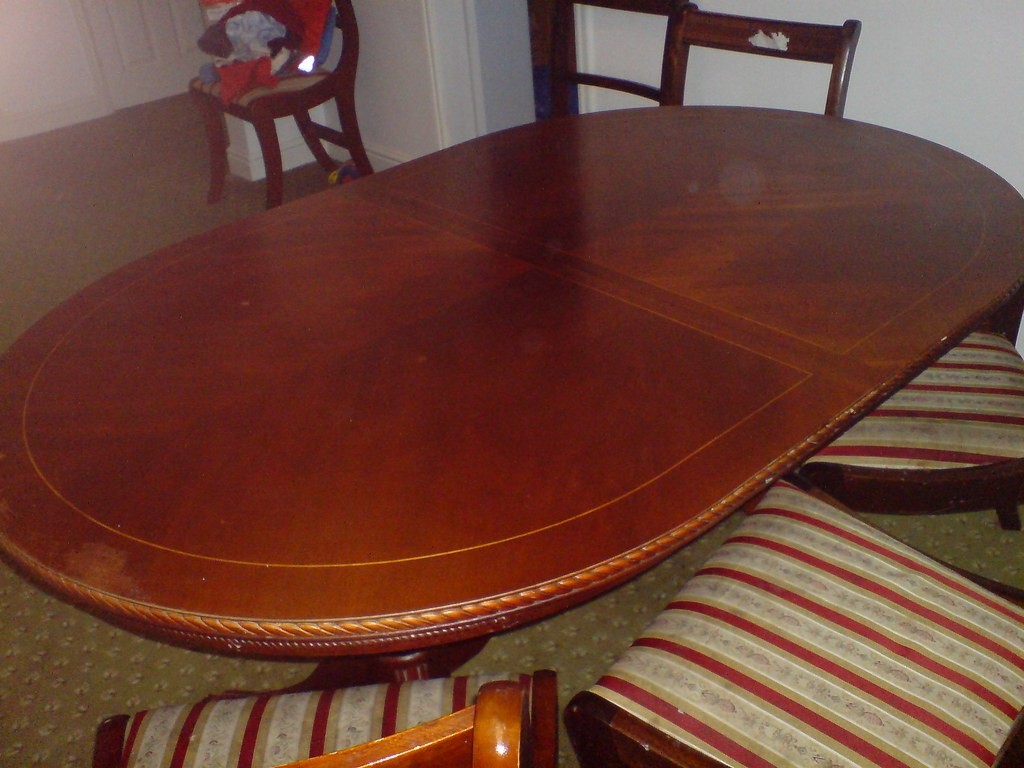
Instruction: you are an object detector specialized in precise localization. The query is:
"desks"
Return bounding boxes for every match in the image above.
[0,106,1024,674]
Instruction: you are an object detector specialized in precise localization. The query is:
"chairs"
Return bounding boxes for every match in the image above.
[91,669,558,768]
[660,4,862,119]
[188,0,375,212]
[563,475,1024,768]
[800,302,1024,530]
[529,0,689,125]
